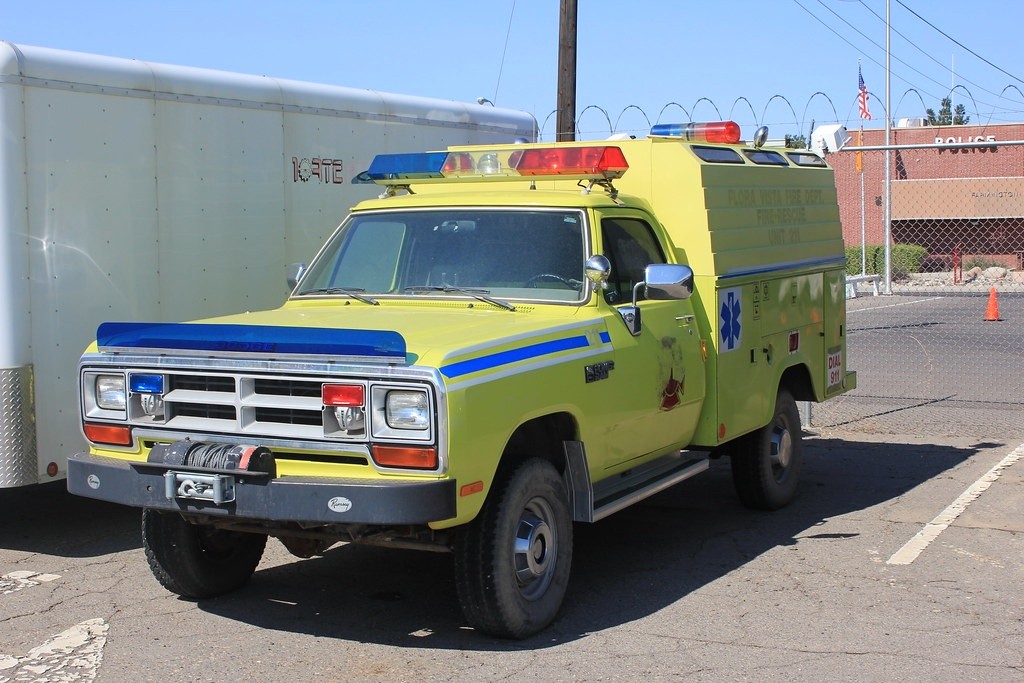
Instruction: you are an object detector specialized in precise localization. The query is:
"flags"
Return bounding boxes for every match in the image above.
[859,66,871,121]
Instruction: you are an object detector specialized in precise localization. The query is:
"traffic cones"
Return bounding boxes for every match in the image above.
[983,287,1001,321]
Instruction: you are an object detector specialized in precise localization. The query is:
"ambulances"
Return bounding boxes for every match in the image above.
[65,120,859,638]
